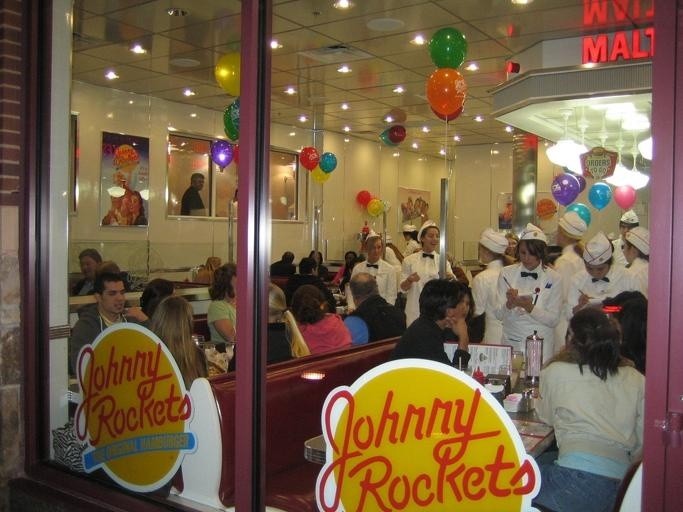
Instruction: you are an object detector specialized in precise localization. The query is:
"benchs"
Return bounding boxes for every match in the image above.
[68,262,645,512]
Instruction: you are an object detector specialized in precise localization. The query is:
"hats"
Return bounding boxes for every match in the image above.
[626,228,649,255]
[403,225,416,232]
[559,212,587,236]
[521,223,546,244]
[620,209,639,223]
[583,232,612,265]
[417,221,439,242]
[365,228,381,240]
[479,229,508,254]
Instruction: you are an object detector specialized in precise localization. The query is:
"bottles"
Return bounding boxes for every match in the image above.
[521,387,538,400]
[187,265,199,283]
[471,364,485,385]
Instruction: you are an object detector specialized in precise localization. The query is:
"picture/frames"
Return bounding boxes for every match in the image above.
[68,108,81,220]
[496,191,513,231]
[396,185,432,235]
[536,190,559,238]
[98,129,151,229]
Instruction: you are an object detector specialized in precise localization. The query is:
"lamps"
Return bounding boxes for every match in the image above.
[547,108,648,190]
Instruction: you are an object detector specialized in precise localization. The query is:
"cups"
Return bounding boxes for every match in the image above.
[490,385,506,408]
[510,351,524,370]
[497,365,510,376]
[225,342,234,360]
[332,292,345,314]
[107,185,125,212]
[190,334,216,371]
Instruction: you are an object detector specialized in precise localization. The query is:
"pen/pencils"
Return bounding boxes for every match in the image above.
[503,277,512,288]
[578,289,584,295]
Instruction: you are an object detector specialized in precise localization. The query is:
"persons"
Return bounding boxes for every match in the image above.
[102,179,147,225]
[473,211,650,375]
[604,290,648,376]
[178,170,205,216]
[332,222,456,328]
[192,257,221,287]
[228,251,407,373]
[72,248,130,296]
[528,307,645,511]
[70,272,150,376]
[206,263,237,343]
[390,280,476,372]
[152,296,207,391]
[140,278,181,327]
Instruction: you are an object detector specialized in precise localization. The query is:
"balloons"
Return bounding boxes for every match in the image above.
[429,28,466,72]
[299,146,319,171]
[429,106,463,123]
[207,50,242,170]
[317,152,337,175]
[380,130,392,144]
[389,124,408,146]
[357,190,372,206]
[367,199,385,219]
[550,171,639,228]
[427,66,468,120]
[311,165,331,185]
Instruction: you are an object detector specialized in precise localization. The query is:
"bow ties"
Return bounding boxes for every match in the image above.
[521,271,538,279]
[367,264,378,269]
[592,277,609,283]
[423,253,434,259]
